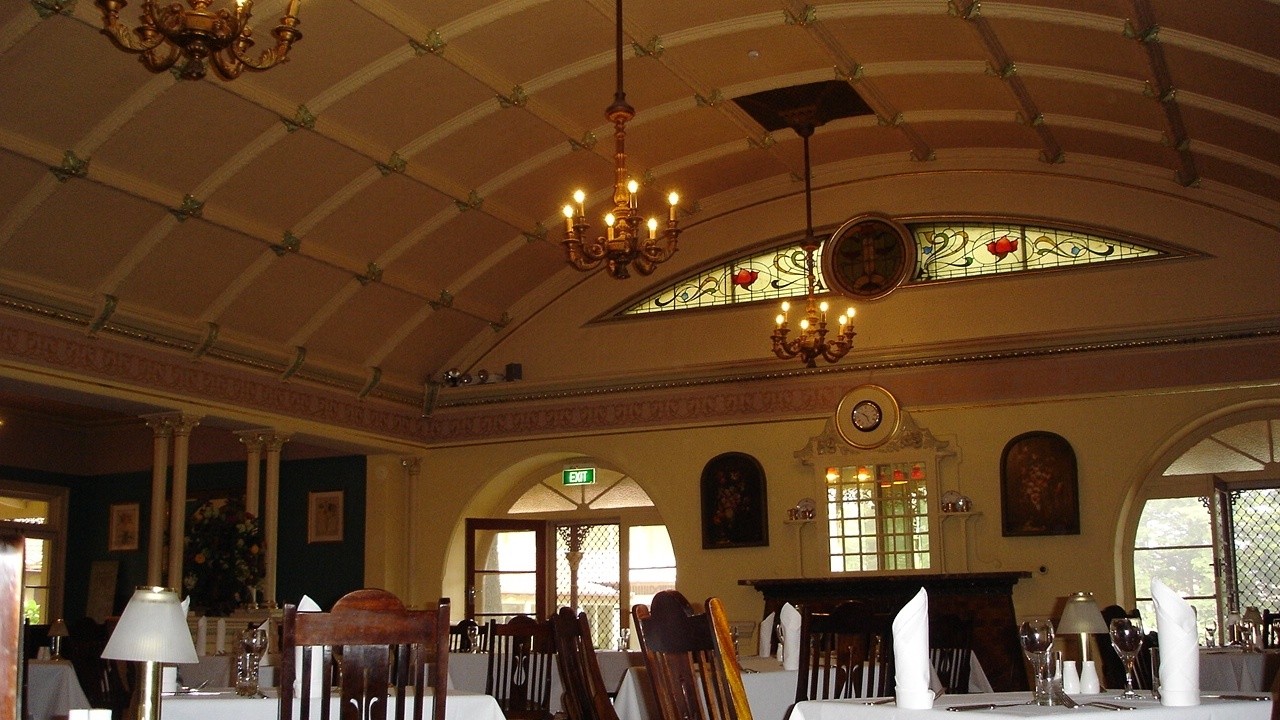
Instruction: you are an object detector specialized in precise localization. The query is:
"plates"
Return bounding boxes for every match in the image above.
[942,490,961,504]
[797,497,816,520]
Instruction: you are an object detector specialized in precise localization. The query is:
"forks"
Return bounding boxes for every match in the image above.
[1054,691,1137,711]
[862,688,946,706]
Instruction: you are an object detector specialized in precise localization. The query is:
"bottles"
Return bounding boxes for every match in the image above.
[38,647,50,660]
[1063,661,1100,694]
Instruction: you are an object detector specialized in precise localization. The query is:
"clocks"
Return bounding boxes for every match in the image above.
[851,400,883,432]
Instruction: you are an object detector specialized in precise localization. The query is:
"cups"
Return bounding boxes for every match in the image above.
[236,652,260,696]
[617,637,626,651]
[1205,631,1214,647]
[1150,647,1160,700]
[729,626,739,644]
[1034,651,1062,706]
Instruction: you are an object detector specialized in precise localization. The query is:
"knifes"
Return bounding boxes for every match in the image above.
[946,704,1028,712]
[1200,695,1270,701]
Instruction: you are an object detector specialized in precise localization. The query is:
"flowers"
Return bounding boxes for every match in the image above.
[161,495,269,592]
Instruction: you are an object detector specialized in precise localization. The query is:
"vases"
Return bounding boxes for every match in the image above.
[244,582,260,610]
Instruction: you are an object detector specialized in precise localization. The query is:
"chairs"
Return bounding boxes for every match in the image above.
[782,598,891,720]
[1262,608,1280,647]
[927,606,980,695]
[482,614,553,720]
[449,617,490,653]
[273,583,454,720]
[631,591,754,720]
[549,606,620,720]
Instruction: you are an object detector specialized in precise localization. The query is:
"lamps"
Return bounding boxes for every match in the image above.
[1244,605,1263,643]
[556,0,683,281]
[1056,589,1109,661]
[1225,610,1242,645]
[463,368,503,385]
[47,617,69,661]
[94,0,303,82]
[100,584,202,720]
[825,463,925,490]
[767,105,858,369]
[442,367,462,388]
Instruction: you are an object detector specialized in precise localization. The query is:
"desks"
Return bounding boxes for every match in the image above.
[448,641,643,714]
[161,679,506,720]
[613,648,886,720]
[789,683,1274,720]
[27,658,93,720]
[1198,643,1280,693]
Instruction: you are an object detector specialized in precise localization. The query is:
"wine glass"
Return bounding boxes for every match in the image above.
[621,628,631,652]
[1206,619,1280,652]
[776,624,784,666]
[1110,617,1144,700]
[240,629,270,699]
[1019,618,1055,705]
[467,626,478,653]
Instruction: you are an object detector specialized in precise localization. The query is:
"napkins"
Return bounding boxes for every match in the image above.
[759,611,775,657]
[259,617,270,666]
[293,592,331,697]
[889,584,936,708]
[778,602,801,672]
[1147,575,1205,707]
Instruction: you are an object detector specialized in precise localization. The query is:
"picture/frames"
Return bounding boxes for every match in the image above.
[107,501,143,555]
[306,486,347,546]
